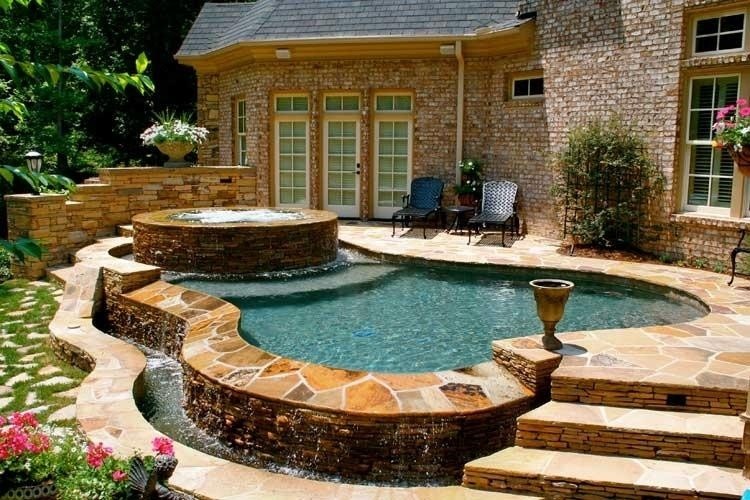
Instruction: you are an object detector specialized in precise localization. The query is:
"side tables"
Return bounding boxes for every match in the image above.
[445,204,480,235]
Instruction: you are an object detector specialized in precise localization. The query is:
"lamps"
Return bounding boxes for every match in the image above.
[22,148,45,194]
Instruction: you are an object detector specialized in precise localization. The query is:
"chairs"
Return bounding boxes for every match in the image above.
[391,177,445,238]
[464,180,521,246]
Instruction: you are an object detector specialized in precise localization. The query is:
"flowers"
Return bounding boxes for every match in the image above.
[710,98,750,153]
[138,105,210,147]
[459,160,477,175]
[453,179,482,194]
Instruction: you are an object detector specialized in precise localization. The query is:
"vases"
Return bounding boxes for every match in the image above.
[726,146,750,177]
[154,141,196,167]
[459,194,480,218]
